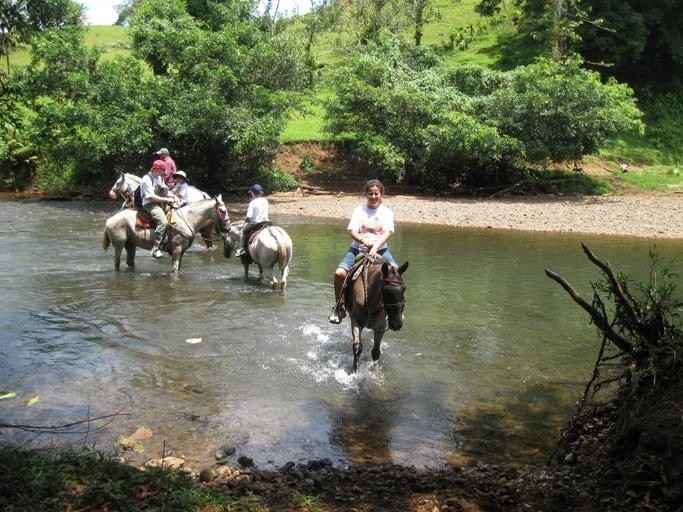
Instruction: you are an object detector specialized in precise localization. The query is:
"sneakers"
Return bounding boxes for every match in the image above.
[234,247,246,256]
[329,305,345,324]
[151,246,163,258]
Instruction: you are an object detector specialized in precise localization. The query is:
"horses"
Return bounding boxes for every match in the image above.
[343,260,408,374]
[103,194,231,270]
[221,220,293,292]
[109,172,214,248]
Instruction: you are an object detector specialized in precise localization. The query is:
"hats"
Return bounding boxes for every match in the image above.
[152,160,166,170]
[172,171,186,179]
[248,184,262,193]
[156,148,169,155]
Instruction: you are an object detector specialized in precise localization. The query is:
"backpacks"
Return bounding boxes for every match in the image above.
[135,174,163,207]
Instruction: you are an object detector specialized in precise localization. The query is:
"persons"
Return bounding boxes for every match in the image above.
[167,171,189,201]
[325,179,407,326]
[139,159,180,261]
[150,148,176,191]
[233,184,270,259]
